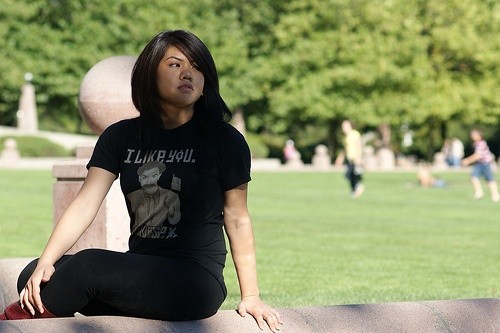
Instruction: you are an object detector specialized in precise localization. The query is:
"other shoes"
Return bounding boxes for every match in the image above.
[0,298,57,320]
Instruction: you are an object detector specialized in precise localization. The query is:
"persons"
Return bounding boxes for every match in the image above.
[0,30,284,333]
[416,137,465,190]
[342,121,364,197]
[283,141,331,169]
[462,129,500,200]
